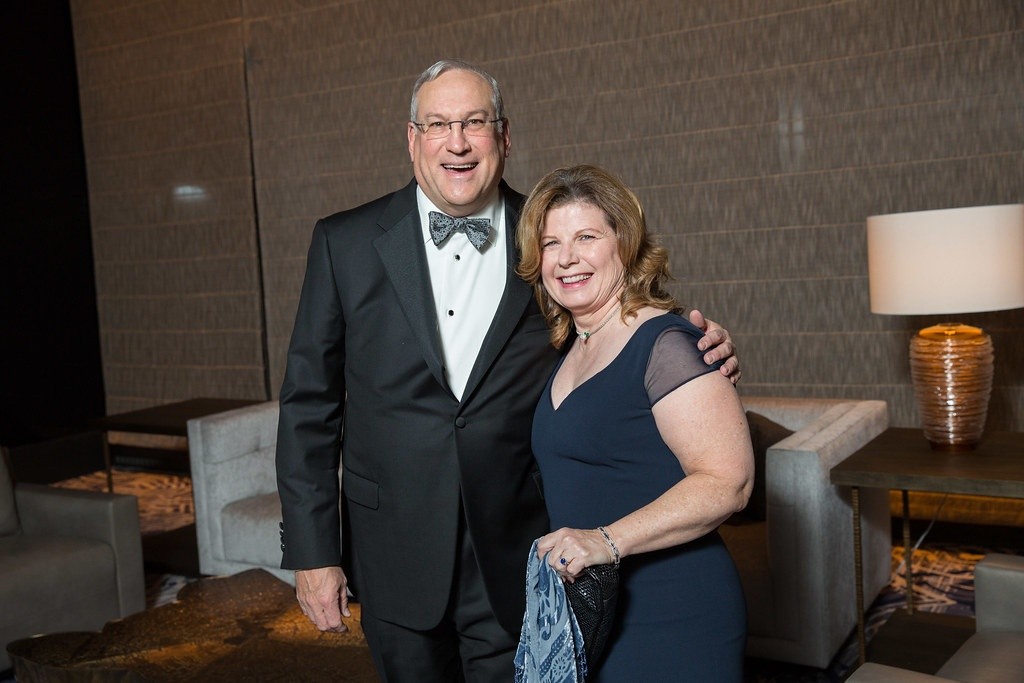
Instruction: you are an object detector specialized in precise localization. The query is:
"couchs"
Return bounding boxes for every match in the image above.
[187,395,892,669]
[0,454,147,672]
[848,553,1024,683]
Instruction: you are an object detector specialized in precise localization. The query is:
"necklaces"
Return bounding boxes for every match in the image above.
[576,305,621,341]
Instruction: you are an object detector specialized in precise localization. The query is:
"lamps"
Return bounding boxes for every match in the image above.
[867,204,1024,447]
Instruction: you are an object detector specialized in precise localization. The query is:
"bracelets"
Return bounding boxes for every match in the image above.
[598,526,620,565]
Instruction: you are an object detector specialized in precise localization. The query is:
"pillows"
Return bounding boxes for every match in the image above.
[723,410,795,527]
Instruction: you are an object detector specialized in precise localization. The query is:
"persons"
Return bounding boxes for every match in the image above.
[274,60,744,683]
[513,164,756,683]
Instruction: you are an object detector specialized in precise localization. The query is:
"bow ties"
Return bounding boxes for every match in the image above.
[428,210,490,250]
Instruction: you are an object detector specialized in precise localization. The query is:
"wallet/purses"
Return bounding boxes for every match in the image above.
[562,564,620,683]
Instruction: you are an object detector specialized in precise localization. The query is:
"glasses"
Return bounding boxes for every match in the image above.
[410,117,503,141]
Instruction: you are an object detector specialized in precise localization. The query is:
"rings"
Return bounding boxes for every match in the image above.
[559,556,567,564]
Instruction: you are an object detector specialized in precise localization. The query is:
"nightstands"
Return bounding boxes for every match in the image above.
[100,397,268,579]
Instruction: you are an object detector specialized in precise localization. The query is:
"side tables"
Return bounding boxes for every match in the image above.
[827,427,1024,675]
